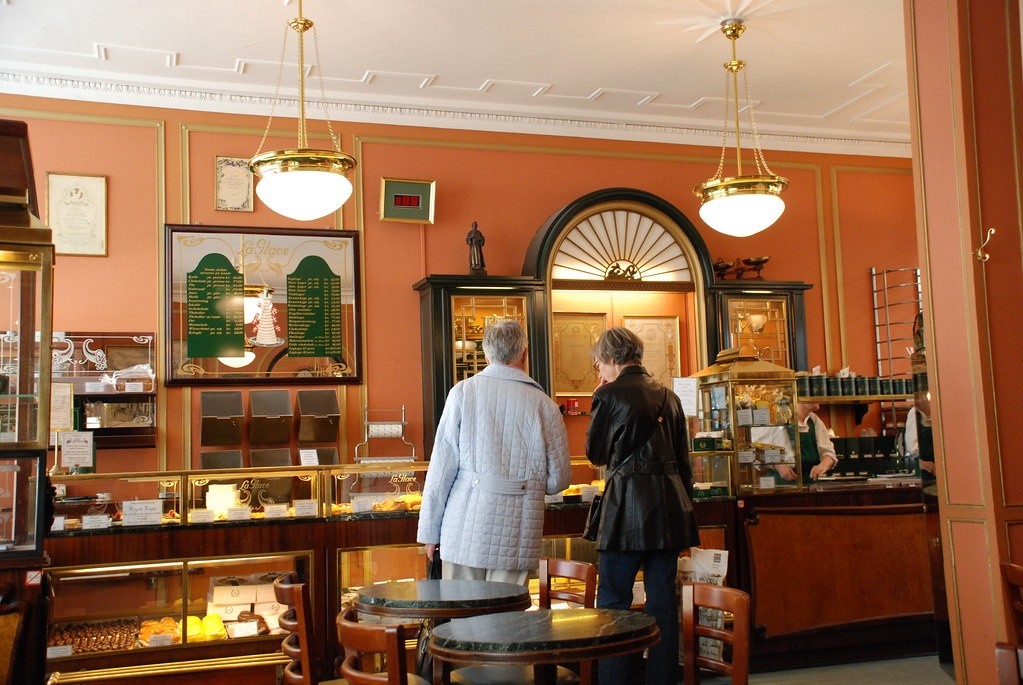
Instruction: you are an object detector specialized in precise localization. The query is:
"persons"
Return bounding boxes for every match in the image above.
[585,327,701,685]
[905,408,921,471]
[751,403,838,485]
[914,383,937,489]
[417,320,574,586]
[465,221,486,268]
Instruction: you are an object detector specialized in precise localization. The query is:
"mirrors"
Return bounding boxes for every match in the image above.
[163,222,365,386]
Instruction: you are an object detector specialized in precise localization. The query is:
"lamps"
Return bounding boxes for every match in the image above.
[692,18,790,238]
[216,234,275,368]
[247,0,357,222]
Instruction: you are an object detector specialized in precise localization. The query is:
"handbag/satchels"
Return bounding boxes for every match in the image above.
[414,547,450,685]
[583,495,602,541]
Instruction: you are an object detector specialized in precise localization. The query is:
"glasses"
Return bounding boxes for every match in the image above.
[593,358,602,372]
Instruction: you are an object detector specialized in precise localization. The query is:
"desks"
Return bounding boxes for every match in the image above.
[426,608,662,685]
[354,579,531,685]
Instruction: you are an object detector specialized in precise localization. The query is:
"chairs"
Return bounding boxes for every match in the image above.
[274,571,432,685]
[682,582,750,685]
[450,556,598,685]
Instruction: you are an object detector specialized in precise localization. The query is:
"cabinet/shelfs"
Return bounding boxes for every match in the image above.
[689,342,804,496]
[412,273,550,458]
[714,280,815,479]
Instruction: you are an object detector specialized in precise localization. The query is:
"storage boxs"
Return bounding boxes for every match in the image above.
[204,572,288,630]
